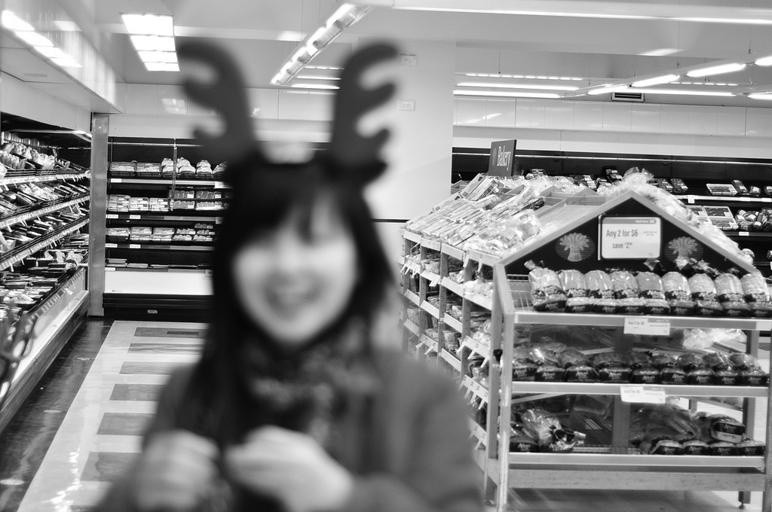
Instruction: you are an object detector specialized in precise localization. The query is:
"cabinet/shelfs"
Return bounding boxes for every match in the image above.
[0,140,92,329]
[106,137,238,297]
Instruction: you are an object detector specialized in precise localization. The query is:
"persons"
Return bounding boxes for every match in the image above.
[90,34,491,511]
